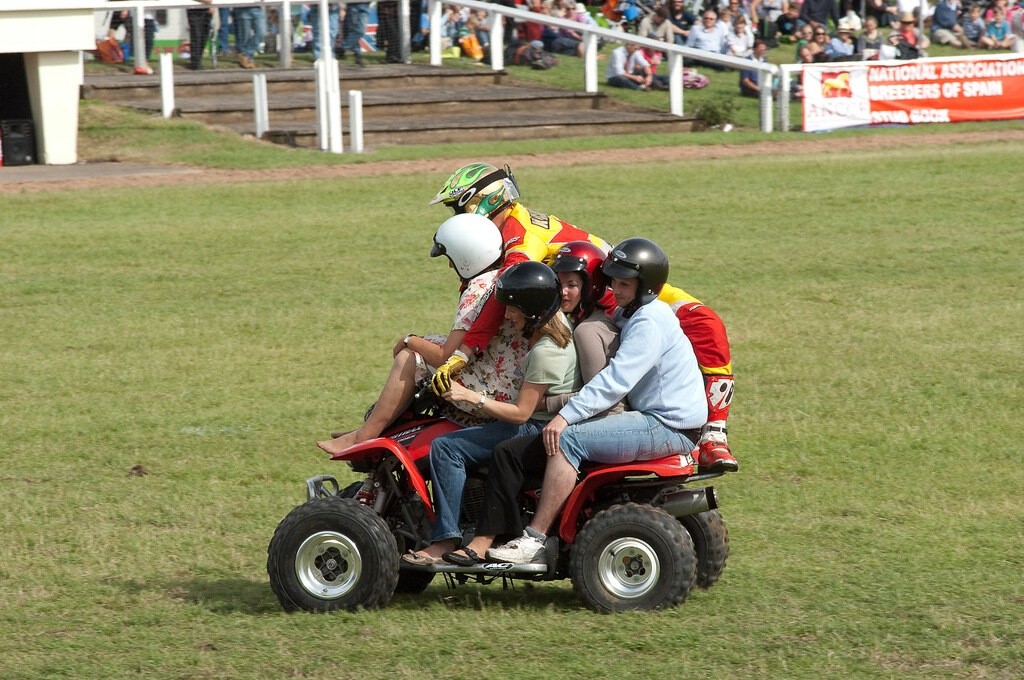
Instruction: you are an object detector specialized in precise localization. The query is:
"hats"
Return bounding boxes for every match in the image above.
[836,23,850,34]
[898,13,916,24]
[574,3,586,14]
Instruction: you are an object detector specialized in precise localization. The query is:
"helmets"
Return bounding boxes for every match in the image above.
[548,242,608,304]
[495,263,562,323]
[429,163,520,218]
[430,214,504,279]
[602,236,669,305]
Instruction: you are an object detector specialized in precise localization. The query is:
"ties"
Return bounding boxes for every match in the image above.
[625,56,630,72]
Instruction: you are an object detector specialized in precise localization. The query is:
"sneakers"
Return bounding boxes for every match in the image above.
[486,531,546,566]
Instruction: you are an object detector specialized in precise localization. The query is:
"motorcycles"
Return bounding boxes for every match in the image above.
[265,379,732,616]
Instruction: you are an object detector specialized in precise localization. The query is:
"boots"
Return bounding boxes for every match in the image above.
[698,375,739,473]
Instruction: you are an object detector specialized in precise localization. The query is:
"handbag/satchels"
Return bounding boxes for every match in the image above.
[96,38,122,63]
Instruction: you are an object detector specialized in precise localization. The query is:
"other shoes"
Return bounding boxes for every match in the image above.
[238,53,257,71]
[402,549,451,565]
[190,62,205,70]
[443,546,483,565]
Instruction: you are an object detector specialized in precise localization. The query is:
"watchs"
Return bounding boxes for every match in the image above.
[404,334,416,347]
[477,396,486,409]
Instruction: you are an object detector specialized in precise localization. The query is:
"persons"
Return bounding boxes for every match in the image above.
[443,241,625,567]
[315,212,530,454]
[109,0,1024,91]
[403,261,582,565]
[486,237,708,568]
[427,162,739,472]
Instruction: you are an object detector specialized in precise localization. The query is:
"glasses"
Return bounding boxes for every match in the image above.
[703,16,715,20]
[814,32,826,37]
[567,7,575,11]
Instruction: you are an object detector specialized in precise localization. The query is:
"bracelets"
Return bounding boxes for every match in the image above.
[452,349,469,364]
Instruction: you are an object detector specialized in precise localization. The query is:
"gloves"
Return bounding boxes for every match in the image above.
[429,354,465,398]
